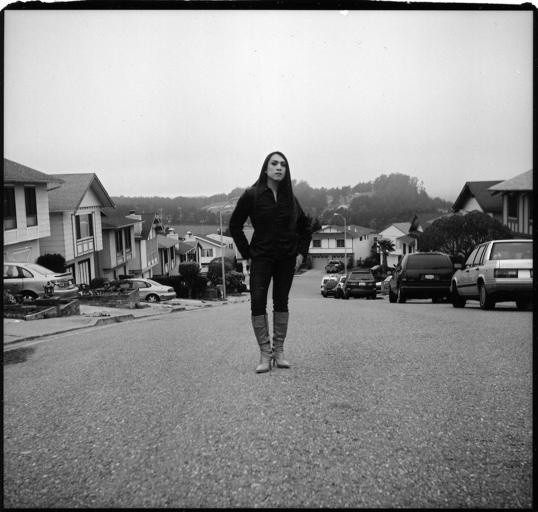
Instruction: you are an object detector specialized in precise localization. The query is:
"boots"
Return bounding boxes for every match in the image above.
[252,315,273,372]
[272,312,290,368]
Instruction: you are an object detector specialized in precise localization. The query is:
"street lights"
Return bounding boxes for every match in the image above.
[334,213,347,275]
[219,196,241,300]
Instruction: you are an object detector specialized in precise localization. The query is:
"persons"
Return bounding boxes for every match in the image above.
[226,150,311,375]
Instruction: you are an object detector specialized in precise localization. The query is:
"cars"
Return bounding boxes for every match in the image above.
[96,277,177,302]
[3,262,78,300]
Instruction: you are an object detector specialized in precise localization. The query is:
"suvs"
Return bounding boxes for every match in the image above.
[320,238,533,312]
